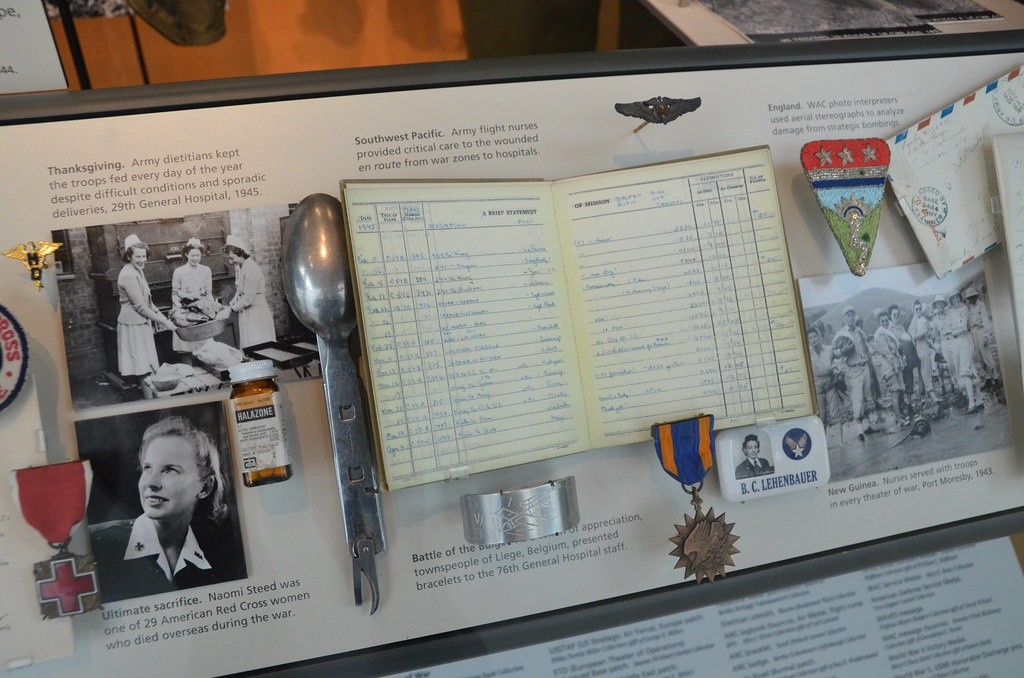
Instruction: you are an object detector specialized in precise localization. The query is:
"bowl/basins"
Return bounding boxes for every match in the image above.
[175,318,224,342]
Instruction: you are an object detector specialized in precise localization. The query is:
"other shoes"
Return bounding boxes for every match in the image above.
[895,414,910,426]
[960,405,979,415]
[864,427,882,435]
[859,433,864,442]
[976,403,984,411]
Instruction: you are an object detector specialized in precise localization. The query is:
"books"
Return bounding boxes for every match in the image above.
[339,144,821,492]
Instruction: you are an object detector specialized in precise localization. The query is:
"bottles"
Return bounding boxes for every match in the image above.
[227,359,292,488]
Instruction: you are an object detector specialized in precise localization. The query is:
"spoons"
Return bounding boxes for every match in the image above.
[280,193,387,615]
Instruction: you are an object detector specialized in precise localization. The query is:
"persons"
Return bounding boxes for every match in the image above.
[223,234,276,351]
[806,286,1000,448]
[169,236,219,368]
[734,434,770,479]
[86,417,248,606]
[116,234,177,399]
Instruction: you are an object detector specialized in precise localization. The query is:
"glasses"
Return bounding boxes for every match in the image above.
[915,308,922,311]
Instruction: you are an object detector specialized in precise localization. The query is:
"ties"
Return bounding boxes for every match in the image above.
[754,460,762,473]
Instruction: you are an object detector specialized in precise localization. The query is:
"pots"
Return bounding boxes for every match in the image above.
[148,363,180,391]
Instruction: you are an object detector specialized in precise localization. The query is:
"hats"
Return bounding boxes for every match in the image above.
[965,288,979,298]
[186,237,204,248]
[932,295,948,308]
[124,233,141,251]
[948,289,962,298]
[842,306,855,316]
[226,234,246,250]
